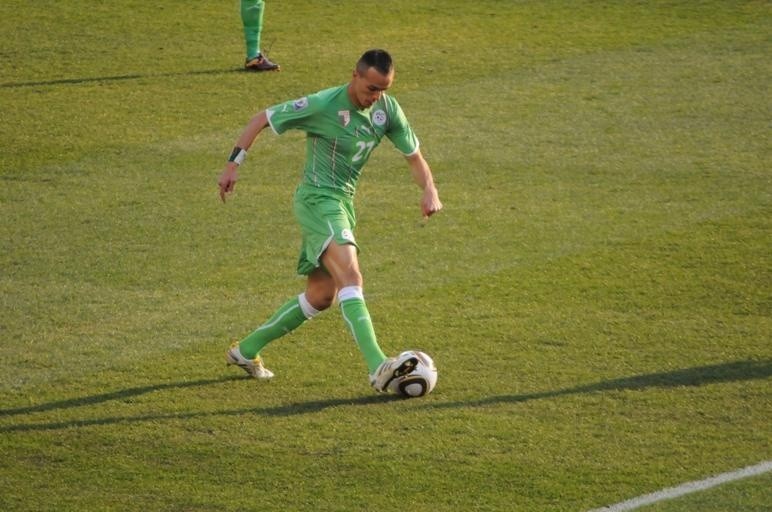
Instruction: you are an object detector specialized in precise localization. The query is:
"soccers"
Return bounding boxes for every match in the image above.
[392,350,438,397]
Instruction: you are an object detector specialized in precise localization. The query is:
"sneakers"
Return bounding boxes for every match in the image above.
[225,342,274,378]
[369,354,418,392]
[245,52,280,71]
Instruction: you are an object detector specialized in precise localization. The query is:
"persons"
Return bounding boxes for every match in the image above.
[214,48,441,392]
[240,0,280,72]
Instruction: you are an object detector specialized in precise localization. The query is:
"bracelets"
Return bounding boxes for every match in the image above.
[227,146,248,166]
